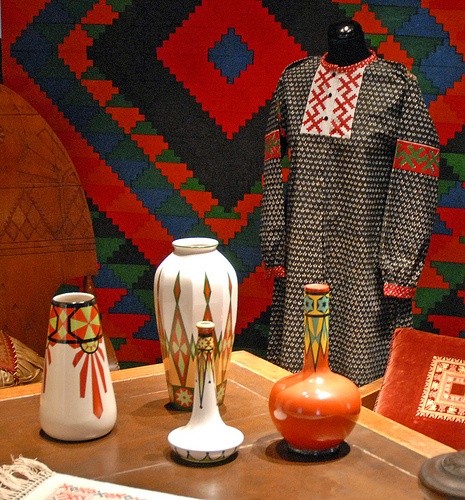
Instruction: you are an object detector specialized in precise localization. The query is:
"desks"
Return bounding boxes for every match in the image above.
[1,348,465,499]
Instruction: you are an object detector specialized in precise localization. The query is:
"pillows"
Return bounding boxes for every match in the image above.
[372,326,465,453]
